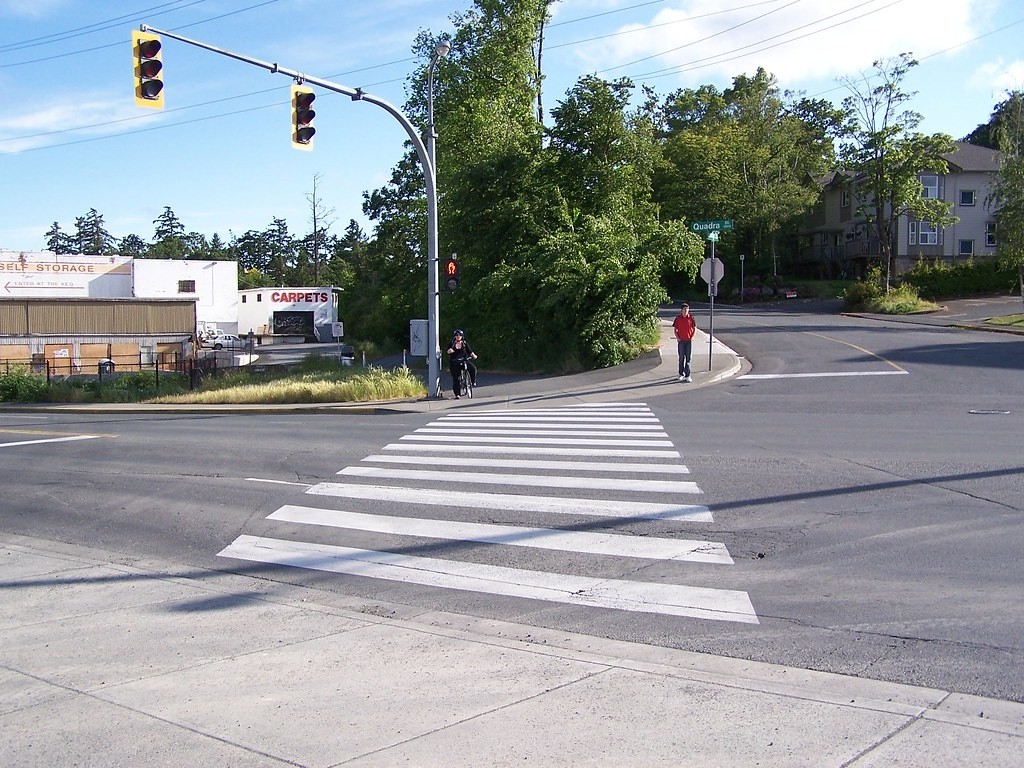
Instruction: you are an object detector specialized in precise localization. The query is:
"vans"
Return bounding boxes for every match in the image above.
[197,328,226,344]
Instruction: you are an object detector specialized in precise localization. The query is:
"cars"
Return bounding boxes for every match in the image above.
[201,333,259,353]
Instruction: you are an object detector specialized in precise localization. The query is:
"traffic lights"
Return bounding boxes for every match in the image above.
[443,258,461,294]
[291,84,317,151]
[131,30,165,109]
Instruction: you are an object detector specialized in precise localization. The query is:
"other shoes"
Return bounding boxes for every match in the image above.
[472,383,476,387]
[685,376,692,383]
[456,394,460,399]
[678,375,683,381]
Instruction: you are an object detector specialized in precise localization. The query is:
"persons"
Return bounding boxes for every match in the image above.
[446,329,477,399]
[672,303,696,383]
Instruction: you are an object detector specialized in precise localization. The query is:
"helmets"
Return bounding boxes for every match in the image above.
[454,330,464,336]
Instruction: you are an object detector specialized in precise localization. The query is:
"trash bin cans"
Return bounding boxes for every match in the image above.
[340,346,355,365]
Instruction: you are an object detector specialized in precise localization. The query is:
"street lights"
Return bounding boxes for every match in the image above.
[426,40,451,397]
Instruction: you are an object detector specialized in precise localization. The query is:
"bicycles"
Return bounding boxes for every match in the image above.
[451,355,475,399]
